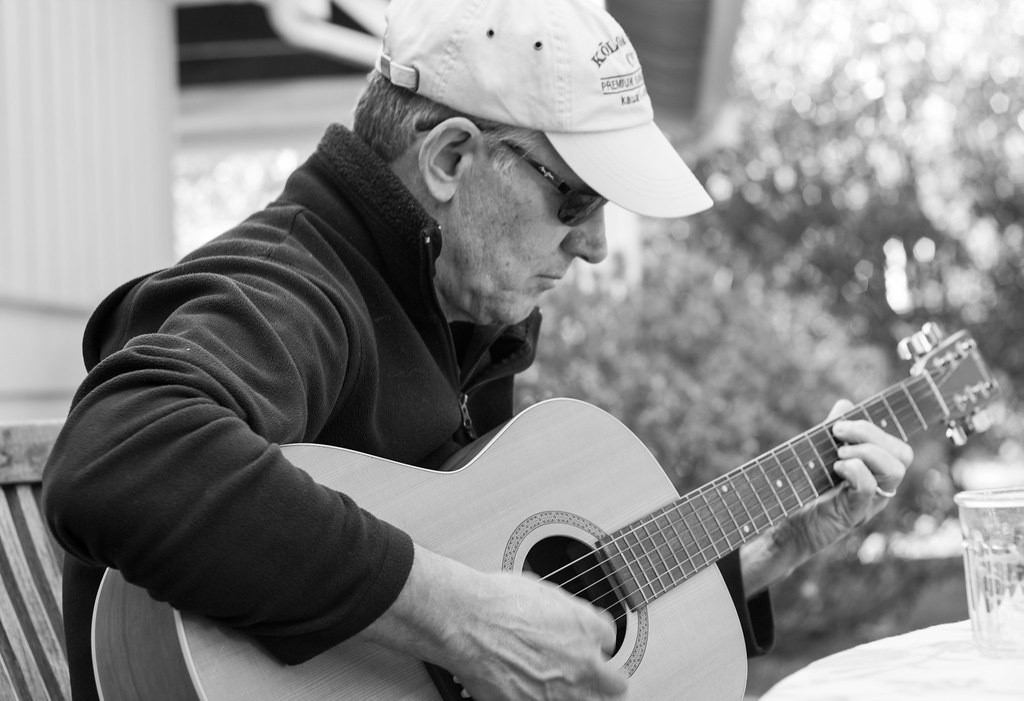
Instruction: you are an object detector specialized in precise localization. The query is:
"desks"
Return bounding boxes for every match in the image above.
[758,620,1024,701]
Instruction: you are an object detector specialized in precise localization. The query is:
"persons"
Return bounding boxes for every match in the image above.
[34,0,915,700]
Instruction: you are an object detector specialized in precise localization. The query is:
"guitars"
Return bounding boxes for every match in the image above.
[90,318,999,700]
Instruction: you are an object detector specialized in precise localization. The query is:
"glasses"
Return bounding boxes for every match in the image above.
[415,119,609,227]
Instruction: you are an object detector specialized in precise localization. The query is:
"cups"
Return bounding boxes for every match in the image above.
[954,486,1024,660]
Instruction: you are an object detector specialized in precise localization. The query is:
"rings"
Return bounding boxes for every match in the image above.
[878,487,896,498]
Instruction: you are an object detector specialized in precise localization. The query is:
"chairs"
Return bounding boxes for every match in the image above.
[0,422,65,701]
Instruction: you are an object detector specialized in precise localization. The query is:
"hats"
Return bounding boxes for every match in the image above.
[375,0,714,219]
